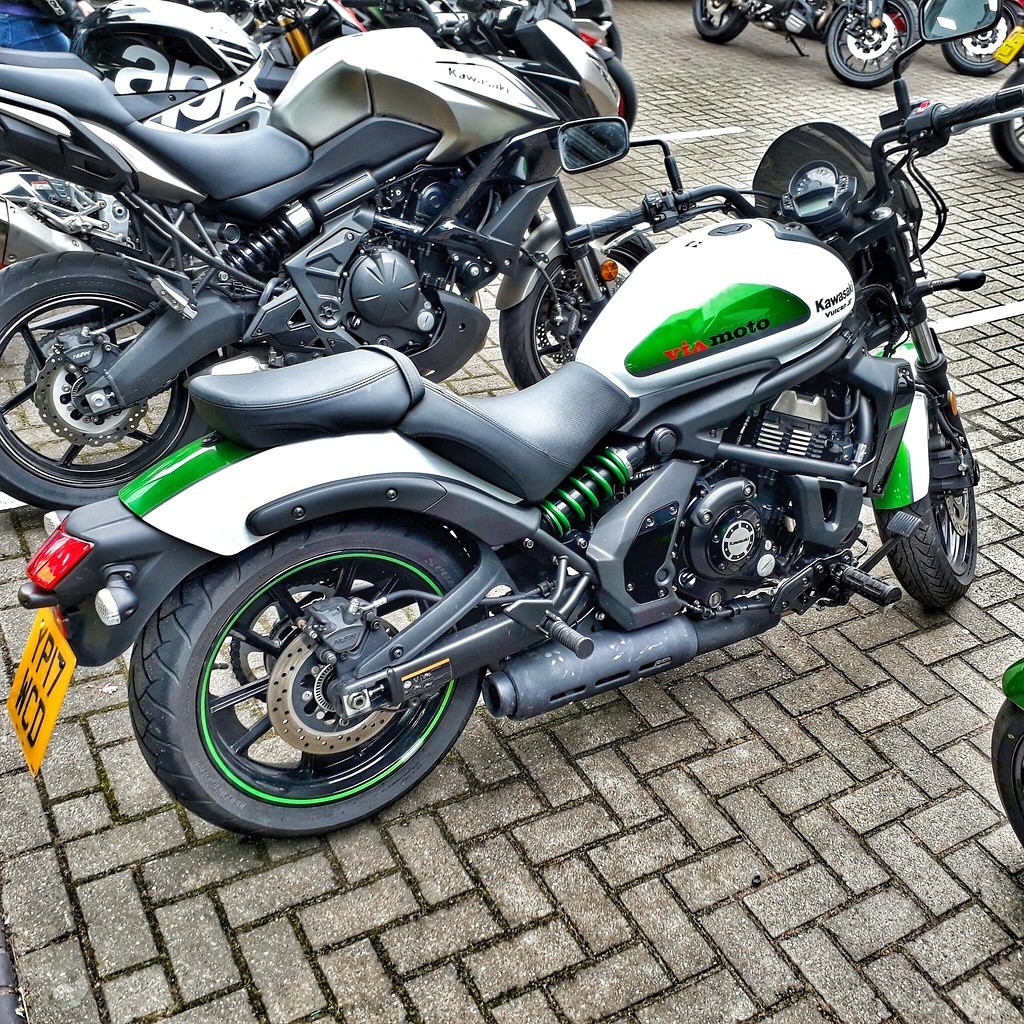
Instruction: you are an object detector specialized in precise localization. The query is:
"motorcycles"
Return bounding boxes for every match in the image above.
[2,2,1024,511]
[4,1,1023,845]
